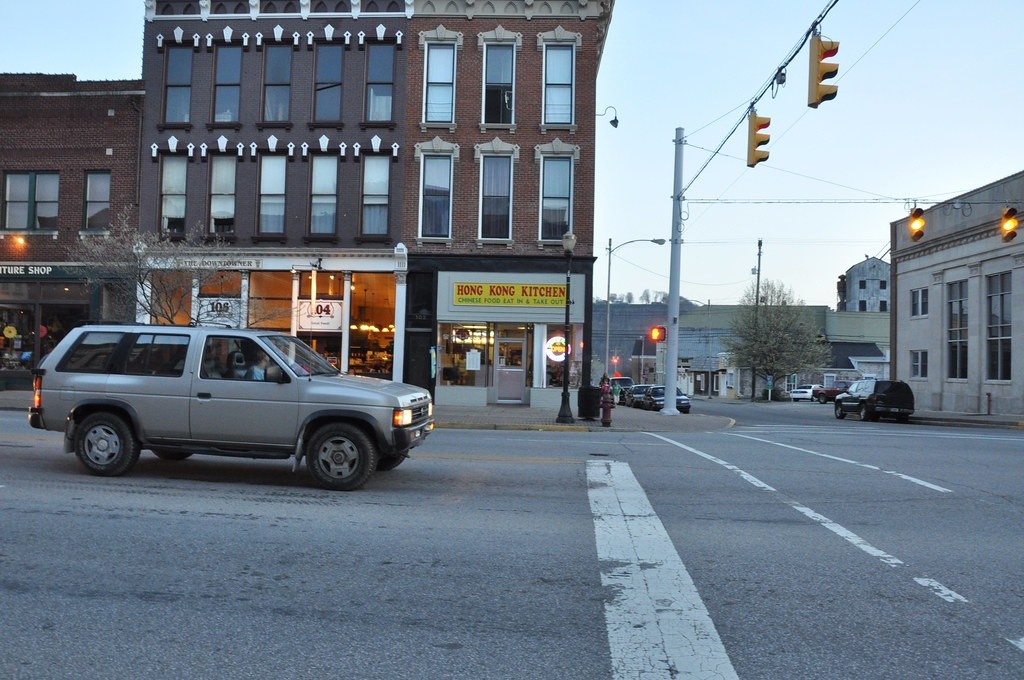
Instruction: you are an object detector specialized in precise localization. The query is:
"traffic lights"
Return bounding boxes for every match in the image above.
[808,37,840,109]
[650,326,666,341]
[1001,207,1018,243]
[747,111,770,168]
[910,208,925,242]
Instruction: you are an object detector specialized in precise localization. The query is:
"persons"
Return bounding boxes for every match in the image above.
[613,380,625,408]
[243,348,270,380]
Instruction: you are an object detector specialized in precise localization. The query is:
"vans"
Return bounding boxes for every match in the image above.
[609,377,635,402]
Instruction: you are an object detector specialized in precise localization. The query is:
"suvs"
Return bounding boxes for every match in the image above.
[27,322,436,491]
[835,377,915,424]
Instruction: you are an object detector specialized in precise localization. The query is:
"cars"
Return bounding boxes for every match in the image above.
[617,385,654,409]
[790,384,824,402]
[641,386,691,413]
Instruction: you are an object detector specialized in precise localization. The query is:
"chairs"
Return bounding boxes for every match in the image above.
[200,355,223,379]
[227,352,249,379]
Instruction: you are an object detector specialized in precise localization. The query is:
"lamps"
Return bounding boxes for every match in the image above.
[351,281,396,333]
[596,106,619,127]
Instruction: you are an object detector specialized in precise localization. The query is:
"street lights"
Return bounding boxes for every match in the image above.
[605,238,666,373]
[751,267,761,400]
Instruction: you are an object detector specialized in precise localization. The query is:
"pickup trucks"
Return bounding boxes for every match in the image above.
[813,380,856,404]
